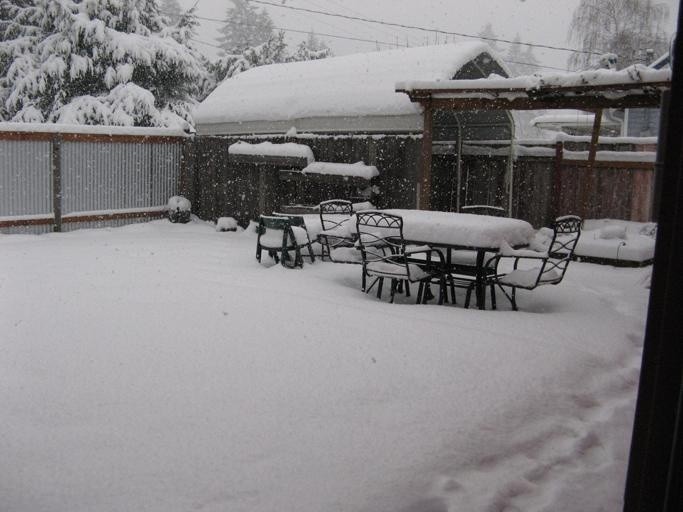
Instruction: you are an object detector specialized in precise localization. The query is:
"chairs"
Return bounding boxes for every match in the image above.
[317,199,583,311]
[255,212,318,269]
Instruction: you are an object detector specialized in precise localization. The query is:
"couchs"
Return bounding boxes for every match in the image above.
[531,218,658,268]
